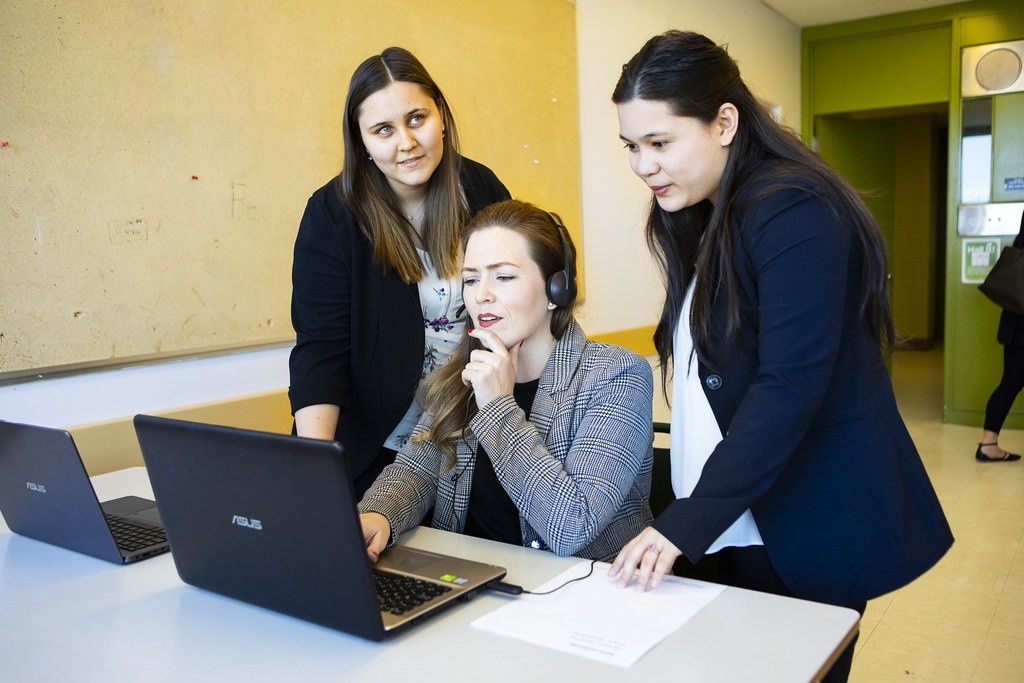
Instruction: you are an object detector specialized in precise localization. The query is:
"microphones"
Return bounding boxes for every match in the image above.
[456,304,466,318]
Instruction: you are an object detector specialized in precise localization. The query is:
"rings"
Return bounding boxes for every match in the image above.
[647,545,660,556]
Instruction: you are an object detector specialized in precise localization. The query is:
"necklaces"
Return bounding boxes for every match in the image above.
[402,214,425,249]
[400,190,429,220]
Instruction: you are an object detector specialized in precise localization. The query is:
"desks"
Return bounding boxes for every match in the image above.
[0,466,861,683]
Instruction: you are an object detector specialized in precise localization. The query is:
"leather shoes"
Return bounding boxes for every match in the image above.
[976,442,1021,462]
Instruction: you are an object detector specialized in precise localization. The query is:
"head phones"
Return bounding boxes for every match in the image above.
[545,211,577,306]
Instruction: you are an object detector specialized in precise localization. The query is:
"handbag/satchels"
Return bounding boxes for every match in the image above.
[978,246,1024,314]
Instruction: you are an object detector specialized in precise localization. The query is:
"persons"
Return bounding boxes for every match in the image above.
[975,209,1024,463]
[613,30,956,683]
[290,46,512,525]
[358,200,655,564]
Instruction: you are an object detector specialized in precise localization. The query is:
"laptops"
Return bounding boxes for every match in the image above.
[0,420,170,564]
[133,414,508,643]
